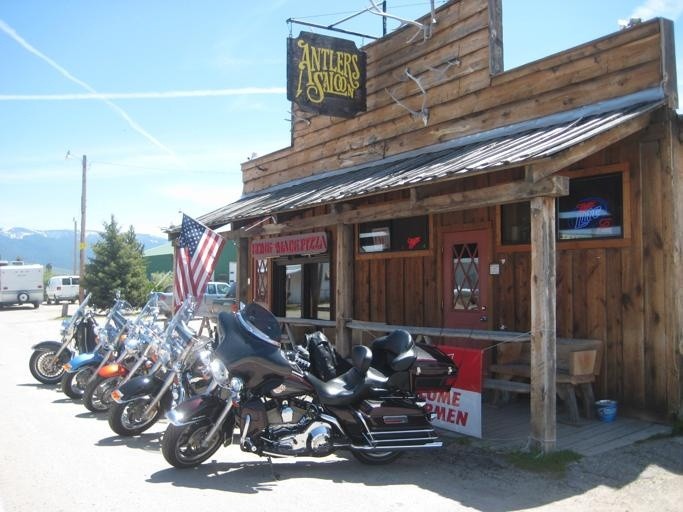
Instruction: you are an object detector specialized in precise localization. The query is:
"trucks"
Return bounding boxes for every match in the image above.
[2,260,46,310]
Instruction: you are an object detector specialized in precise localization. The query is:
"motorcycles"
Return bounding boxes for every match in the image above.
[161,299,459,468]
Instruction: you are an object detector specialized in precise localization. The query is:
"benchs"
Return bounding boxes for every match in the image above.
[489,338,602,426]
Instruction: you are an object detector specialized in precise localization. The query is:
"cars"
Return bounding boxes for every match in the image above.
[160,281,232,318]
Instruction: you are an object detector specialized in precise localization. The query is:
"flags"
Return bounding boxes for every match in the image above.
[173,213,227,319]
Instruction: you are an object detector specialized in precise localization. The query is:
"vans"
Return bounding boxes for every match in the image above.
[46,274,88,306]
